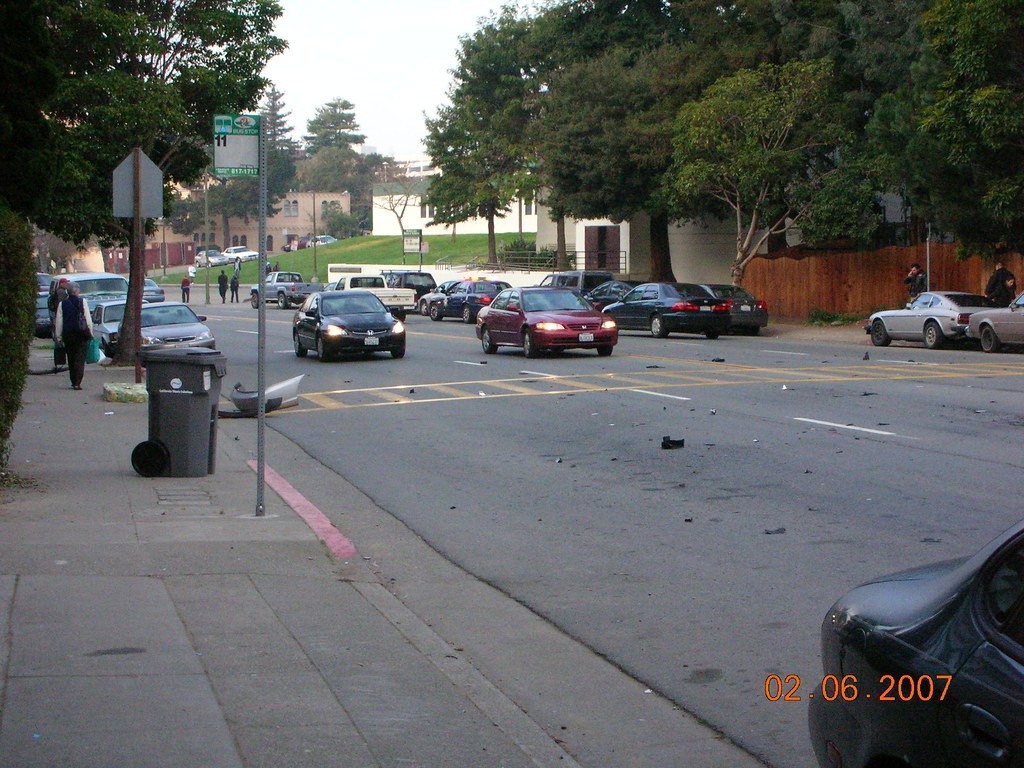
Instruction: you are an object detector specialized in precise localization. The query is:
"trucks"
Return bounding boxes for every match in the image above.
[333,274,417,323]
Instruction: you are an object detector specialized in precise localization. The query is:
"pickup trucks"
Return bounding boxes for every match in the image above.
[249,271,325,310]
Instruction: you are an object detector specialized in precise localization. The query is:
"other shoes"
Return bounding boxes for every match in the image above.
[71,384,82,390]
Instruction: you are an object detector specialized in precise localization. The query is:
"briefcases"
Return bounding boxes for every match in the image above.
[85,338,99,364]
[54,344,66,365]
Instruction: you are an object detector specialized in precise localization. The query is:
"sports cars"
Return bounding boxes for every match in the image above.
[863,290,1004,349]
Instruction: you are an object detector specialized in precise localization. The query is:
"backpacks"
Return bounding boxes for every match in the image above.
[48,288,65,312]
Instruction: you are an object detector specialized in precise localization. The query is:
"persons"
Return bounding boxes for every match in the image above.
[188,264,196,284]
[904,264,927,303]
[266,262,271,274]
[230,276,239,302]
[234,259,241,279]
[56,282,94,389]
[218,270,228,303]
[181,276,190,304]
[273,262,280,271]
[54,278,70,371]
[984,262,1017,307]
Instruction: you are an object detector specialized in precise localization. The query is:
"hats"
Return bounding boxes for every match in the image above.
[60,278,70,283]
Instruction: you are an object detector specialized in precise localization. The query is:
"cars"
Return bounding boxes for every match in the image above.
[306,235,338,249]
[963,290,1024,353]
[291,290,407,363]
[320,282,339,293]
[118,302,216,352]
[697,283,769,337]
[194,249,230,269]
[808,516,1024,768]
[89,299,151,359]
[125,278,165,302]
[32,272,59,340]
[416,279,515,323]
[222,245,259,263]
[475,286,620,359]
[583,280,649,312]
[600,282,732,341]
[280,237,313,253]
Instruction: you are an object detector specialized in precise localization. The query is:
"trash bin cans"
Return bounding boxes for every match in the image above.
[130,340,225,477]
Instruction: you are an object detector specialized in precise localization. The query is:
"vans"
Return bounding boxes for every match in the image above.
[373,272,438,314]
[540,270,614,298]
[47,272,134,328]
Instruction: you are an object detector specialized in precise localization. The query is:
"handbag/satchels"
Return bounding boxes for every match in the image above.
[79,298,86,332]
[97,349,106,364]
